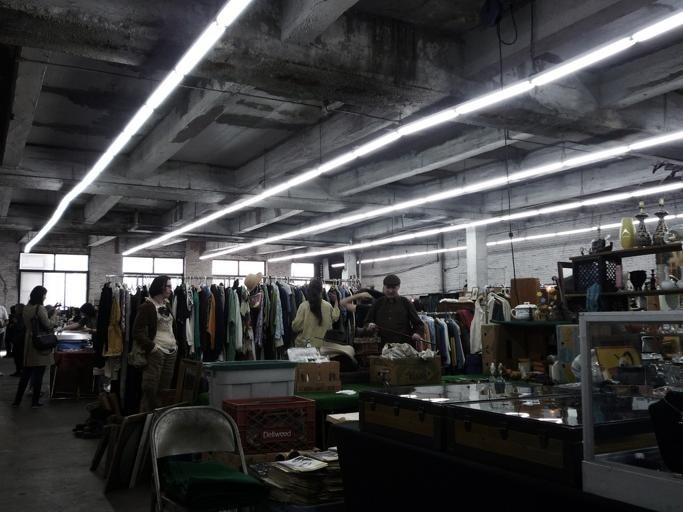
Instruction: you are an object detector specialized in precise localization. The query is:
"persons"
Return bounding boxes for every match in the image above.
[6,285,101,409]
[342,290,378,365]
[292,280,340,348]
[366,275,427,347]
[133,277,179,412]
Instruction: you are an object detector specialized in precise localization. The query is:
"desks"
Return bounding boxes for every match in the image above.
[48,348,98,402]
[355,379,666,512]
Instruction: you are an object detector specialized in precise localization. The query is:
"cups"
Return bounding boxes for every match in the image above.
[666,365,683,387]
[477,379,490,396]
[376,368,390,388]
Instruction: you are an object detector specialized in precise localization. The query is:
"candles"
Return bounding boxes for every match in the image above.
[638,200,644,208]
[657,197,664,205]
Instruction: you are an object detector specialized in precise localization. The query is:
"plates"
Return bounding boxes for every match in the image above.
[570,348,603,385]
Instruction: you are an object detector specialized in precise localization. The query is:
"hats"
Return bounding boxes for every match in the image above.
[244,271,264,292]
[329,355,361,373]
[383,275,401,287]
[314,328,349,346]
[320,342,358,362]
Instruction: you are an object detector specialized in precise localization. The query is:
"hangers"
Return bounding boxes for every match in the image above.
[103,273,362,295]
[417,310,456,321]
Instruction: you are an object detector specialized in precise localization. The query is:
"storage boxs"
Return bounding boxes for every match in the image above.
[178,348,443,449]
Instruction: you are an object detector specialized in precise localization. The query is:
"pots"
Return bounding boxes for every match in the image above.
[509,301,537,320]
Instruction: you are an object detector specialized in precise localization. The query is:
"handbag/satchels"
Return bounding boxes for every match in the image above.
[128,326,150,368]
[32,334,58,352]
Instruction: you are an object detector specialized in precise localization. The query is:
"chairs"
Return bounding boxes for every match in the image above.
[329,421,470,512]
[147,400,269,512]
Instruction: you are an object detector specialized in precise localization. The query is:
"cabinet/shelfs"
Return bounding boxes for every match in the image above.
[559,234,682,308]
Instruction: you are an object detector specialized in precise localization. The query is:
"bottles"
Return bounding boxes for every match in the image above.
[518,358,530,375]
[653,198,669,245]
[644,266,682,291]
[635,197,652,246]
[488,362,506,395]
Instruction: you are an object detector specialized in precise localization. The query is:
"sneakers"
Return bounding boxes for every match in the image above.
[11,402,20,408]
[9,371,22,377]
[32,402,44,409]
